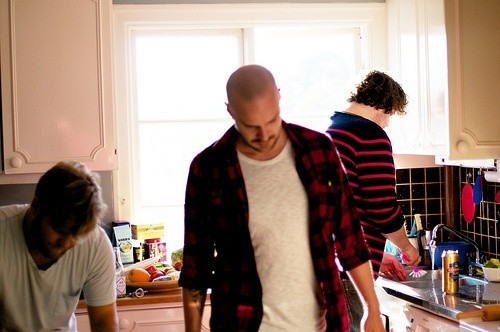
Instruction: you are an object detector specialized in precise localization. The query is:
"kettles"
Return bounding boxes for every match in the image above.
[415,223,434,266]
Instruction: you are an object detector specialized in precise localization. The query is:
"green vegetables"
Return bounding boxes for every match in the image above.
[483,260,500,268]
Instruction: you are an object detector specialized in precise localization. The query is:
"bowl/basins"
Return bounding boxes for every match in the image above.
[482,266,500,282]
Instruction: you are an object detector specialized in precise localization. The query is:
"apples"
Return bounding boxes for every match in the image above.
[129,268,151,282]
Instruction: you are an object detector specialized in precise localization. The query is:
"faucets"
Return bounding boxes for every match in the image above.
[429,224,480,264]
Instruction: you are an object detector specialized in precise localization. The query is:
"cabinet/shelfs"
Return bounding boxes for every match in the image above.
[0,0,119,184]
[75,299,211,332]
[384,0,500,161]
[377,291,475,332]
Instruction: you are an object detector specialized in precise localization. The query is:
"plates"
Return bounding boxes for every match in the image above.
[126,281,182,292]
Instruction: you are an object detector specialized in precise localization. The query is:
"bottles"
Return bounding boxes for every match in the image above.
[113,247,127,298]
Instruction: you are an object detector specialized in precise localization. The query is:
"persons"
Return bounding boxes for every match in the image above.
[0,161,119,332]
[325,69,422,332]
[178,65,384,332]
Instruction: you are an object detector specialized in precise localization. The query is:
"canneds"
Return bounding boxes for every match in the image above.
[132,246,144,263]
[441,250,459,294]
[443,294,457,309]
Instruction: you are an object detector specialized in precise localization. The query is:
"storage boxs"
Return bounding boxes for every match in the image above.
[144,242,166,262]
[131,224,165,240]
[112,221,134,263]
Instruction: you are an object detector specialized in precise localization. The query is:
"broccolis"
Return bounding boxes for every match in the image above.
[170,248,182,271]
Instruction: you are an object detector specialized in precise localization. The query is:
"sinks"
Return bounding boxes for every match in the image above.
[378,269,488,292]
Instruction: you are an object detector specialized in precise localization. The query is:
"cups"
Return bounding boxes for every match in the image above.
[400,232,419,266]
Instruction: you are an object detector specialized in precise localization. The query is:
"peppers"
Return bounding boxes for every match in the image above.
[146,265,164,280]
